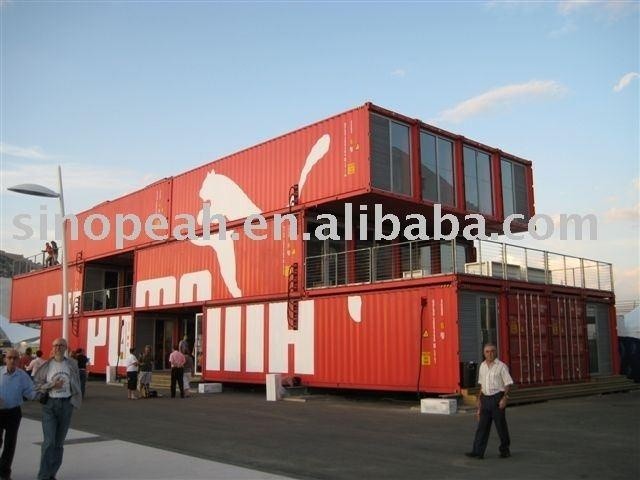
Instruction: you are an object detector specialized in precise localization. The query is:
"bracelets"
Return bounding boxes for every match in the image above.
[503,392,510,399]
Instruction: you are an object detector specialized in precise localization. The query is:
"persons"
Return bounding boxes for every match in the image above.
[167,345,186,399]
[0,346,89,402]
[465,340,514,459]
[178,333,188,354]
[138,344,154,398]
[31,338,84,480]
[182,349,194,393]
[41,242,53,268]
[0,349,65,480]
[125,347,144,400]
[51,240,58,266]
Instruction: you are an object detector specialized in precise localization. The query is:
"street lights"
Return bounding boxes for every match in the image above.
[7,164,72,361]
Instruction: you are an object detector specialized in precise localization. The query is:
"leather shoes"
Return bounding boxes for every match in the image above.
[465,453,483,459]
[497,454,510,458]
